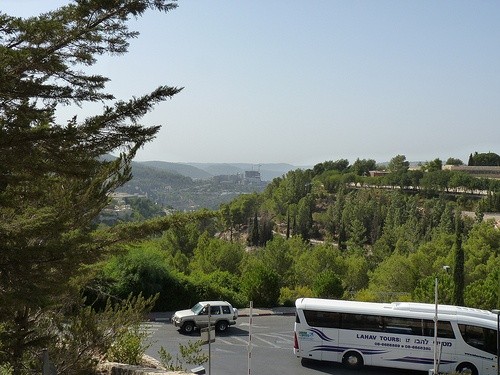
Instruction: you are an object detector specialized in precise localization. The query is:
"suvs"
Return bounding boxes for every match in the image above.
[171,300,238,334]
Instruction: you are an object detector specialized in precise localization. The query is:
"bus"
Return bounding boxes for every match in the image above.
[293,297,500,375]
